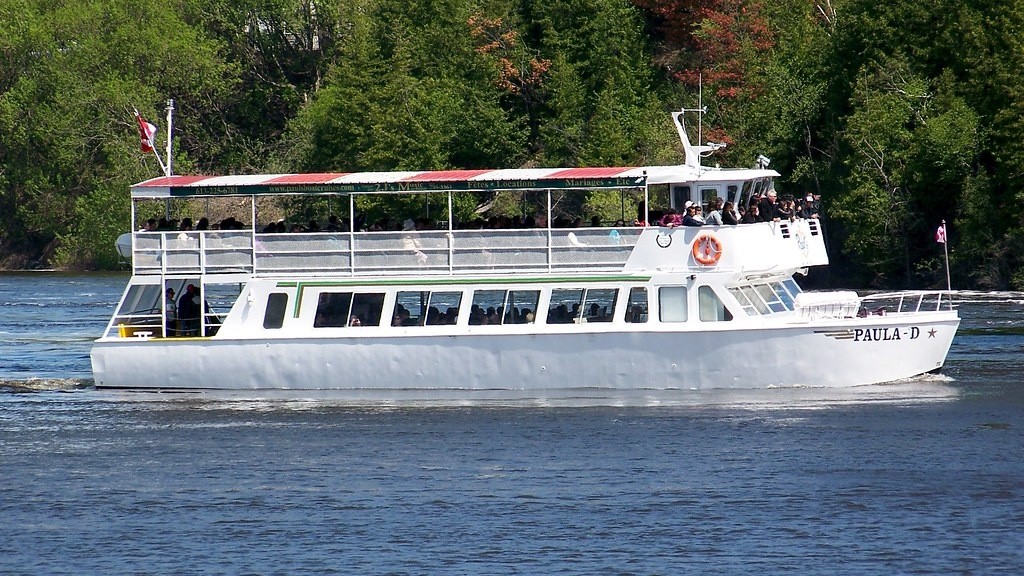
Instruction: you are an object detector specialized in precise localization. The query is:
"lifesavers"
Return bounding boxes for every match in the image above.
[692,234,722,265]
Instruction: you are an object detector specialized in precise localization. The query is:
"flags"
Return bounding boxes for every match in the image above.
[935,226,945,243]
[136,115,157,152]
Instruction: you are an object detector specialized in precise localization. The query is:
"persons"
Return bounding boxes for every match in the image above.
[145,217,243,231]
[591,302,600,316]
[613,220,625,227]
[315,303,579,327]
[660,208,683,229]
[738,189,819,223]
[590,216,601,227]
[722,200,738,225]
[705,198,723,225]
[178,284,208,337]
[682,200,705,227]
[456,215,584,230]
[262,216,432,233]
[166,287,177,338]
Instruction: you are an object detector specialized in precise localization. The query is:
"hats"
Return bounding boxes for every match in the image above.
[806,196,813,201]
[685,201,693,209]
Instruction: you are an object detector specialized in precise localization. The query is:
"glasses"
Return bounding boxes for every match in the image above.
[395,318,400,320]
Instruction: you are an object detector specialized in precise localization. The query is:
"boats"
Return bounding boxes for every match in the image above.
[88,71,961,390]
